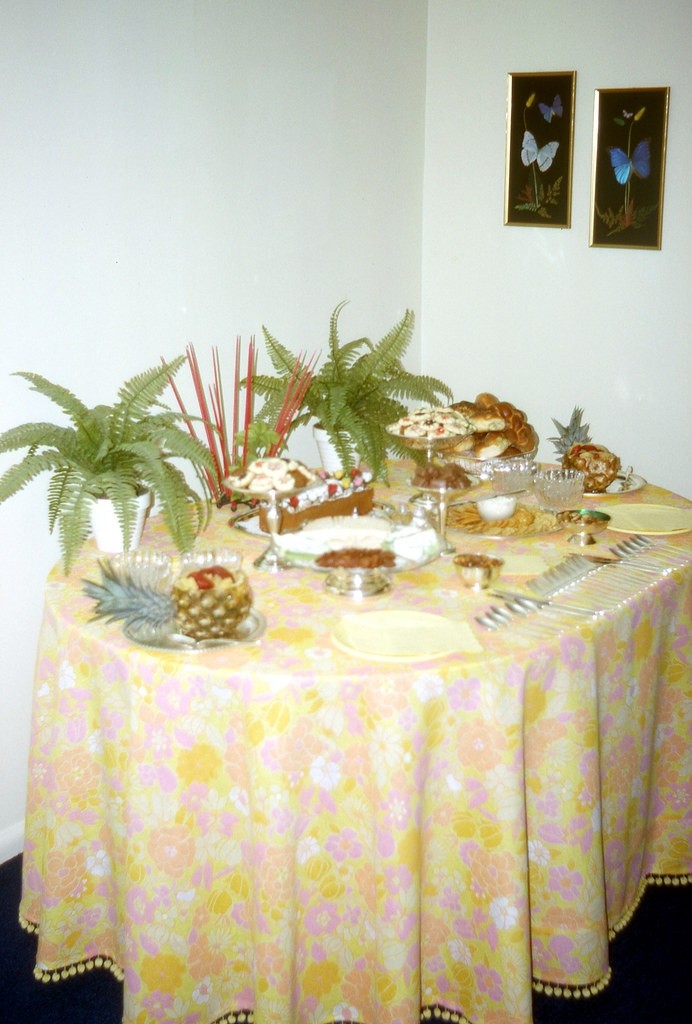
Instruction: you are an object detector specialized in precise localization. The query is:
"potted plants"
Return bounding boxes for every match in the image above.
[236,298,452,491]
[0,354,224,578]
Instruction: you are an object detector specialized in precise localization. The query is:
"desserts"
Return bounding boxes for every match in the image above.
[390,406,471,437]
[412,462,471,489]
[229,458,375,533]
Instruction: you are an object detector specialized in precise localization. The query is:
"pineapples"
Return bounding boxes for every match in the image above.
[78,559,253,640]
[547,405,621,491]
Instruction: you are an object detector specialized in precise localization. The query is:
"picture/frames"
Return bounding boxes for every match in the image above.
[589,87,670,250]
[503,71,578,229]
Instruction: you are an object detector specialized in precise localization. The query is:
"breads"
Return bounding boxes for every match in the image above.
[441,392,536,460]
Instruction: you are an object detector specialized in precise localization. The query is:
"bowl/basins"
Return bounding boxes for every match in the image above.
[453,552,505,590]
[533,468,585,514]
[476,496,518,520]
[438,445,538,480]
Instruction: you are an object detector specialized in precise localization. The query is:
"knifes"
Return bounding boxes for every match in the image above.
[564,552,669,577]
[487,589,604,621]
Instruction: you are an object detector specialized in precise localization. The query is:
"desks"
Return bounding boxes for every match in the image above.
[19,463,692,1024]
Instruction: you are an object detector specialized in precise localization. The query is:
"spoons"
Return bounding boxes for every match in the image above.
[474,596,591,644]
[608,534,692,573]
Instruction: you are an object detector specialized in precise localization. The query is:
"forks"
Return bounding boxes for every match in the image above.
[524,554,659,616]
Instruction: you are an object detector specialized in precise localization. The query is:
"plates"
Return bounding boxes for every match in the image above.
[582,469,648,498]
[121,607,266,655]
[229,500,396,541]
[595,504,692,535]
[445,500,566,539]
[330,610,461,665]
